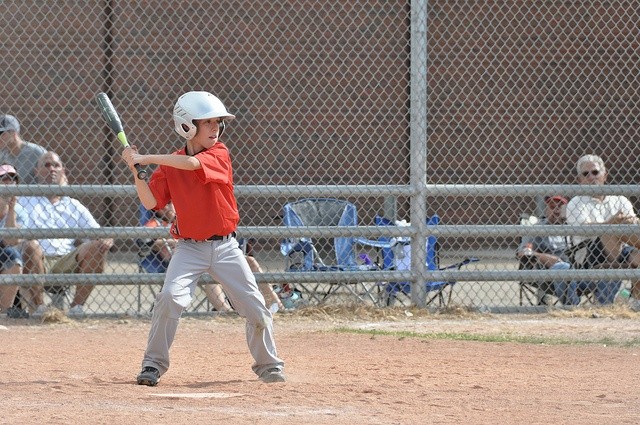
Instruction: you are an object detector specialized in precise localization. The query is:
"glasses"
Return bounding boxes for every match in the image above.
[583,170,598,176]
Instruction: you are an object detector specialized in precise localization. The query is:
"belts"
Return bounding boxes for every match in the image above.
[207,232,235,240]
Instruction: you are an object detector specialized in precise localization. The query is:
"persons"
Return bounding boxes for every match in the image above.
[0,163,25,318]
[122,91,294,385]
[140,198,294,316]
[566,154,640,313]
[0,114,47,185]
[520,195,589,309]
[18,151,114,317]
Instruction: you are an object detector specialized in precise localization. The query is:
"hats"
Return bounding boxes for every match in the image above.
[0,164,17,176]
[0,114,20,131]
[545,195,571,204]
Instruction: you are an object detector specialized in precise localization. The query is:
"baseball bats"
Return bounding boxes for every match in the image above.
[95,92,147,180]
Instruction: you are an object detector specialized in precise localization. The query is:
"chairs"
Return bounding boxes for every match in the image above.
[283,200,392,306]
[373,215,479,307]
[517,216,596,305]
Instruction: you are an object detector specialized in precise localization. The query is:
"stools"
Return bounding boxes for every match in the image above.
[137,205,216,314]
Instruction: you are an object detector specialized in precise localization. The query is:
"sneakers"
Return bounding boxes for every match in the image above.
[69,304,87,314]
[137,367,161,385]
[260,368,285,382]
[37,304,46,312]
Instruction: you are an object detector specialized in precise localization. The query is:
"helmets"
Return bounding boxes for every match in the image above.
[173,91,236,140]
